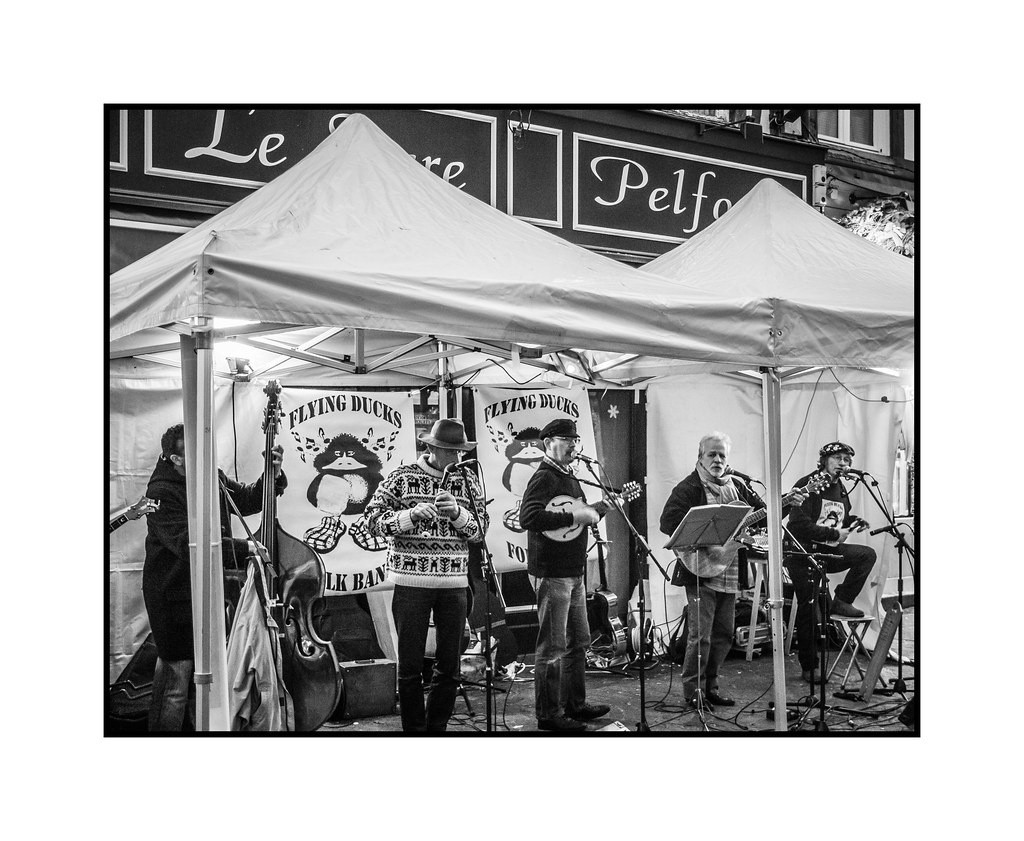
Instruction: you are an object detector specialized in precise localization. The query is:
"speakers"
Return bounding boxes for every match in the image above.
[333,660,397,720]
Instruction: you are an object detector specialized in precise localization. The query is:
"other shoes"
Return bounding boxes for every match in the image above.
[830,595,864,617]
[803,668,827,684]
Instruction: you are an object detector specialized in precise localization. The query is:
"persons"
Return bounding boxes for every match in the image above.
[362,416,489,734]
[517,419,625,732]
[783,441,877,684]
[141,420,288,734]
[660,432,810,714]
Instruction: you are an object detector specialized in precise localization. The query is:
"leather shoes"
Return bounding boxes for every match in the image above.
[565,702,609,720]
[538,716,587,731]
[706,695,735,706]
[687,697,714,711]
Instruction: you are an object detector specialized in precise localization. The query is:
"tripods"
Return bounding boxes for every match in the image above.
[744,474,916,720]
[648,505,754,732]
[423,655,506,715]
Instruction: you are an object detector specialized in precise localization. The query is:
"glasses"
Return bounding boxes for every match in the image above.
[832,455,851,464]
[557,438,580,444]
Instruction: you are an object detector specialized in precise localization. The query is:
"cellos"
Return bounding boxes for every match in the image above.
[224,381,346,733]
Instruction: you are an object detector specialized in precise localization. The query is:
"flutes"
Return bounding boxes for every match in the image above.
[430,473,453,528]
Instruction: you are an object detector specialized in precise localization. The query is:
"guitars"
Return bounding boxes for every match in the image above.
[586,523,628,661]
[541,480,643,543]
[673,471,833,581]
[110,494,163,534]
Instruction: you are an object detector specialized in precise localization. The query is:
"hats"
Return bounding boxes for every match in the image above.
[821,442,855,454]
[417,418,478,451]
[539,418,581,438]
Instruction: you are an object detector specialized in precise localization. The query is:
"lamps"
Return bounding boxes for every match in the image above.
[849,191,914,215]
[694,115,763,142]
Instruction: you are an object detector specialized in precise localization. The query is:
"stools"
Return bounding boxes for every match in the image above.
[825,613,887,690]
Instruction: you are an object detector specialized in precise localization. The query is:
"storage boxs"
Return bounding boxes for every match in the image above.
[339,658,397,719]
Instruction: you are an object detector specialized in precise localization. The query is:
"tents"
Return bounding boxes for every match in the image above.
[107,113,914,733]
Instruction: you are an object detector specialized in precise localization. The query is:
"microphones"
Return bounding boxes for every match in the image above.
[447,460,477,473]
[737,539,763,548]
[843,466,869,475]
[725,467,761,483]
[570,450,599,464]
[870,523,904,536]
